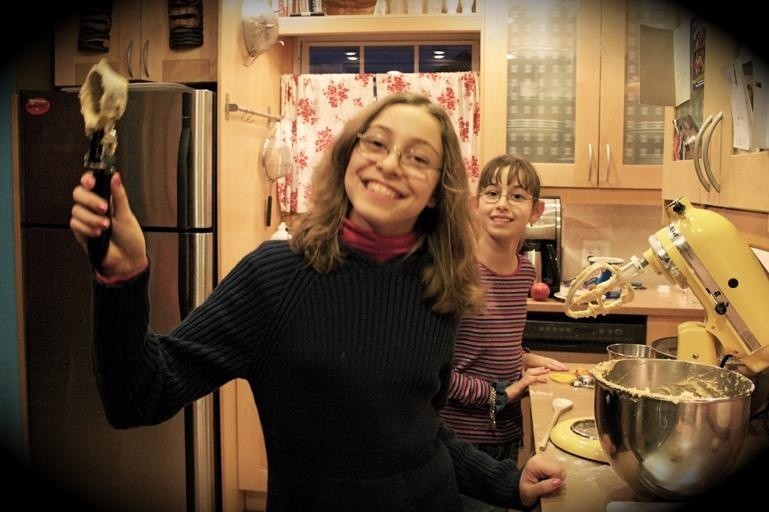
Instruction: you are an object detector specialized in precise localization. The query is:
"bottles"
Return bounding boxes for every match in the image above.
[521,243,541,290]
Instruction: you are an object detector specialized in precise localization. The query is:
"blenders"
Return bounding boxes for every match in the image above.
[562,196,769,500]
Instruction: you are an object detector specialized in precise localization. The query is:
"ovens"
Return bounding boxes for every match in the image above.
[519,311,648,351]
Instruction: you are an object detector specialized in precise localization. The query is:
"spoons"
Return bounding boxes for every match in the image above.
[569,379,595,389]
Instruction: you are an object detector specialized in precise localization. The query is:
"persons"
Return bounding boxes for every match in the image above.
[439,149,570,466]
[66,87,569,512]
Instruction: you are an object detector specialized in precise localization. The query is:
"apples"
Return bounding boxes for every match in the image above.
[531,282,550,300]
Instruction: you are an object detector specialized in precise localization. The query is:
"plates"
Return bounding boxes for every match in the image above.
[553,288,607,302]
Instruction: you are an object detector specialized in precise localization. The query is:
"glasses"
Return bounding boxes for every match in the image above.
[478,190,533,205]
[357,133,442,177]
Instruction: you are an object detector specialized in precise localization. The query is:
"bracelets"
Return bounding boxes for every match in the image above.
[486,385,499,423]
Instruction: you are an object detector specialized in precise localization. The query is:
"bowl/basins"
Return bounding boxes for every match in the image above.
[608,335,698,360]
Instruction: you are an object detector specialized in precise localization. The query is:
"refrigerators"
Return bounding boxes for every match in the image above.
[5,85,222,512]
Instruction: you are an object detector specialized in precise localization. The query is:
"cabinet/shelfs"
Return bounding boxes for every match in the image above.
[663,6,769,214]
[484,0,662,191]
[53,0,219,87]
